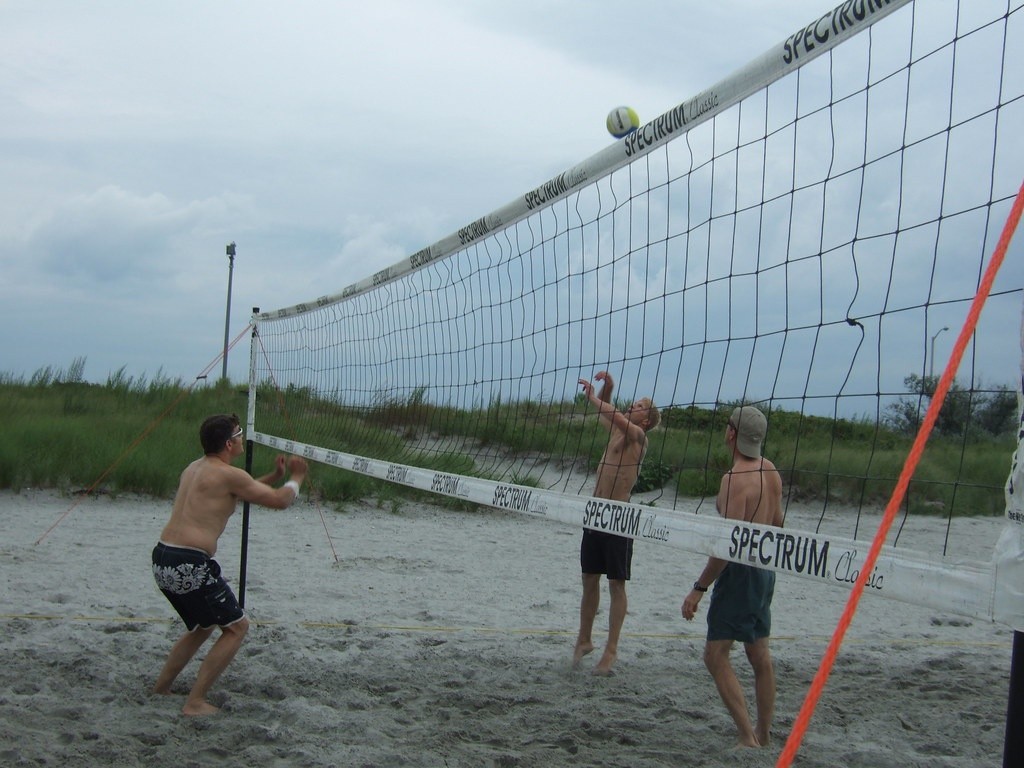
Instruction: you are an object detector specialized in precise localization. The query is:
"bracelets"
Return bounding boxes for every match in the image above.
[283,481,300,497]
[694,582,707,592]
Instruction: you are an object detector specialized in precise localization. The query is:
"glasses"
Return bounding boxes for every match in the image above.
[727,418,737,432]
[224,428,243,447]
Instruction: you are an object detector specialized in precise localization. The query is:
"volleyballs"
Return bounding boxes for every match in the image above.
[605,104,640,140]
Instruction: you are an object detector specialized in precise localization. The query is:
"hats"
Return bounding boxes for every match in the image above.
[732,406,766,459]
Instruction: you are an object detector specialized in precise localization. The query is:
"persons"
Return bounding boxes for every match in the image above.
[573,371,659,675]
[682,405,784,747]
[152,416,308,715]
